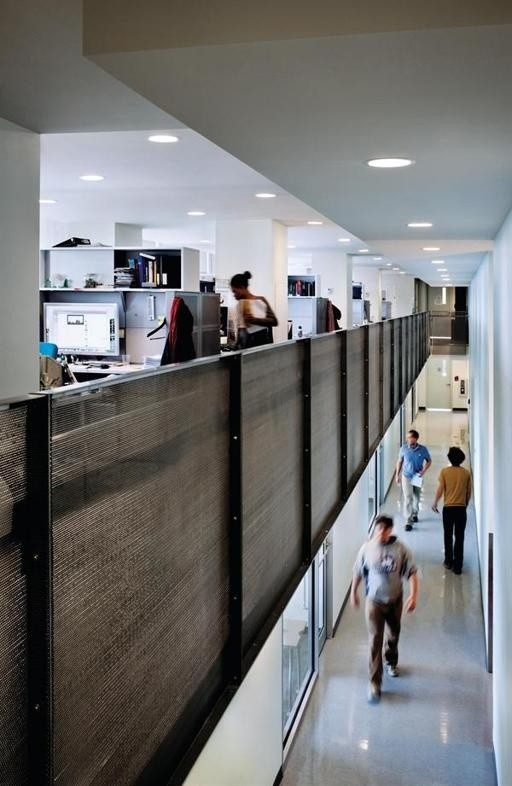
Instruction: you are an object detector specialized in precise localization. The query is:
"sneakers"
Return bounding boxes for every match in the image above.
[386,663,400,677]
[411,517,418,522]
[371,684,381,702]
[405,525,412,531]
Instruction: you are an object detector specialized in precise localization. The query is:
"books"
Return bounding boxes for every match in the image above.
[288,278,316,296]
[114,252,180,289]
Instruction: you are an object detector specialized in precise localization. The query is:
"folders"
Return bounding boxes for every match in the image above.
[53,236,90,249]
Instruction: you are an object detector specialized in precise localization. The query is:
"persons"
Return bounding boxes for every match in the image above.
[393,429,432,532]
[226,271,279,351]
[348,512,421,705]
[430,446,474,577]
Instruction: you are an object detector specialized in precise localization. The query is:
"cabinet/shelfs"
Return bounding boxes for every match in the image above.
[288,274,320,338]
[39,247,200,375]
[351,282,364,326]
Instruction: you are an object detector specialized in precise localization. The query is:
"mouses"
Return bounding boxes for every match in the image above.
[101,364,110,369]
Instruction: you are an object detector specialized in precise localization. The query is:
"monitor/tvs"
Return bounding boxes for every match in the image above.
[43,303,120,357]
